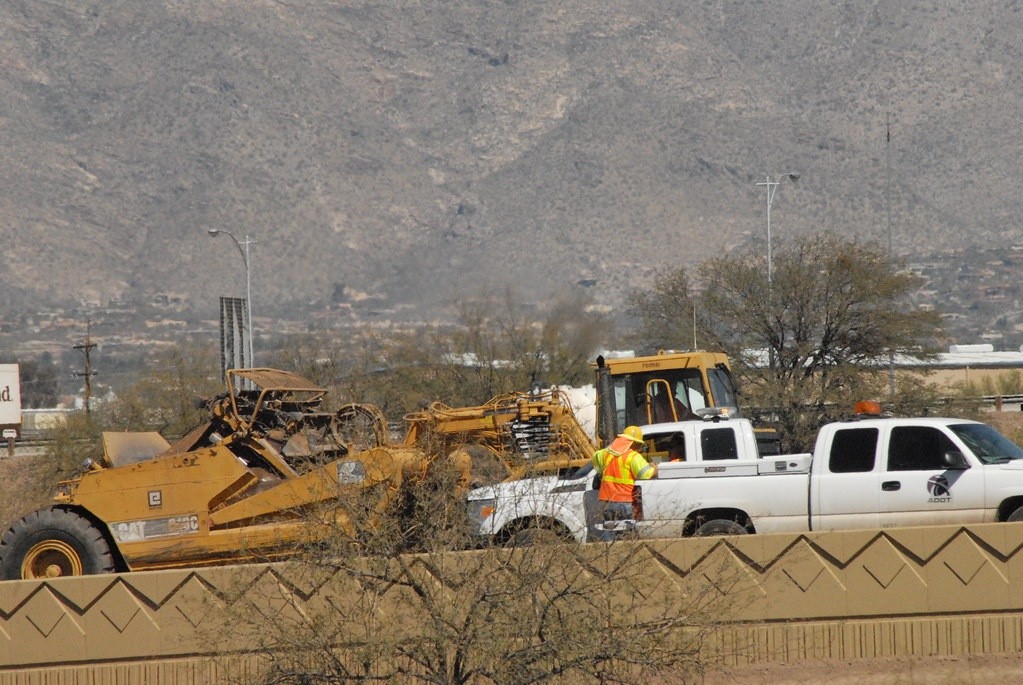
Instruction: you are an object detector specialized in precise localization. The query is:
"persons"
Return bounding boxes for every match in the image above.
[668,437,685,461]
[577,425,658,541]
[652,379,699,422]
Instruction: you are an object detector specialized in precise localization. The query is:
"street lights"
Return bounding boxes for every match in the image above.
[755,170,802,325]
[208,227,254,391]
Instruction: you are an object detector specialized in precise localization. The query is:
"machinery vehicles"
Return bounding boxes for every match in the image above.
[0,349,780,580]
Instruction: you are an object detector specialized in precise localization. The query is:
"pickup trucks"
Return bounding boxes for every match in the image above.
[466,407,814,548]
[629,418,1023,540]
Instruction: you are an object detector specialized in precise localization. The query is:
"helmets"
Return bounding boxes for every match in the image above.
[617,426,645,443]
